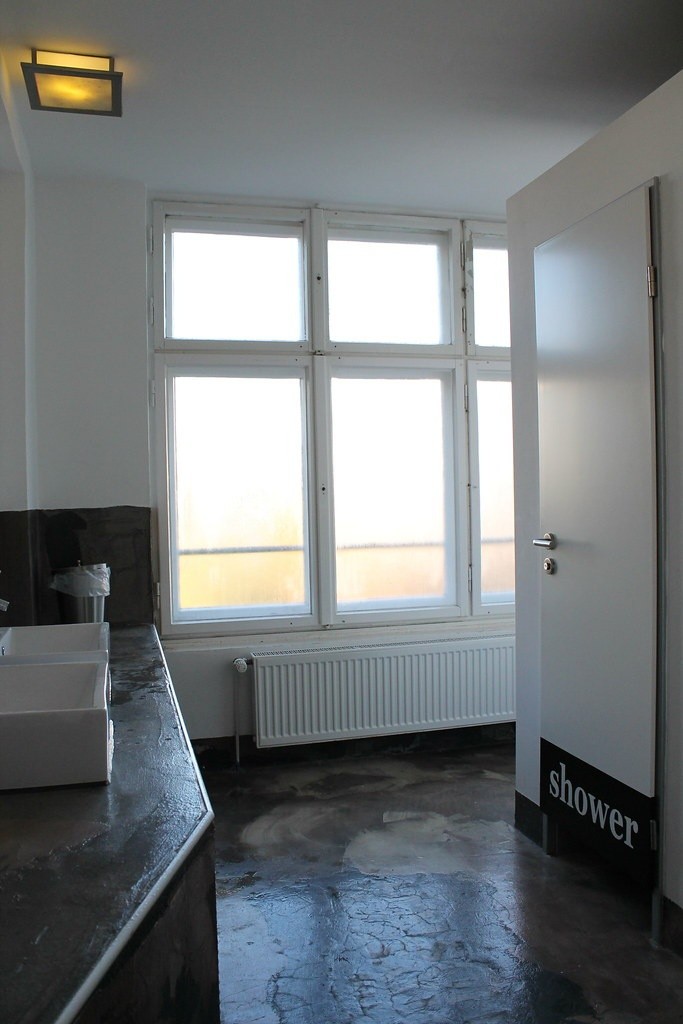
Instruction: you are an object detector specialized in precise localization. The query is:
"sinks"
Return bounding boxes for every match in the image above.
[0,622,111,658]
[0,658,111,789]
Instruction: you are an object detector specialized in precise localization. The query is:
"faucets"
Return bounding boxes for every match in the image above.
[0,599,10,612]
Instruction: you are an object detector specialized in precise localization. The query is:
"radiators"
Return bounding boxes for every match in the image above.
[251,635,517,750]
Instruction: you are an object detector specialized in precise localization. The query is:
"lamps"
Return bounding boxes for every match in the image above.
[21,48,124,118]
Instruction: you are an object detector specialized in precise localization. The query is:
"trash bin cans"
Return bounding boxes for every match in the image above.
[52,562,110,624]
